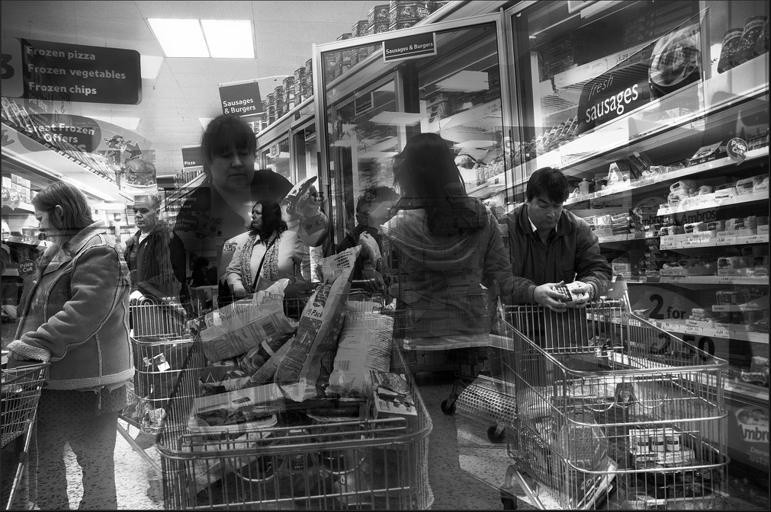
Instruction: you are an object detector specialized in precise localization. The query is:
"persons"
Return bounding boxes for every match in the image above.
[119,193,187,450]
[335,185,403,297]
[357,132,516,511]
[6,178,136,512]
[224,197,305,299]
[494,163,616,389]
[167,111,335,341]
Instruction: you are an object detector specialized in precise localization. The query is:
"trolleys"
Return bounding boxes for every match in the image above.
[157,276,438,512]
[0,349,48,509]
[490,296,733,512]
[124,290,203,437]
